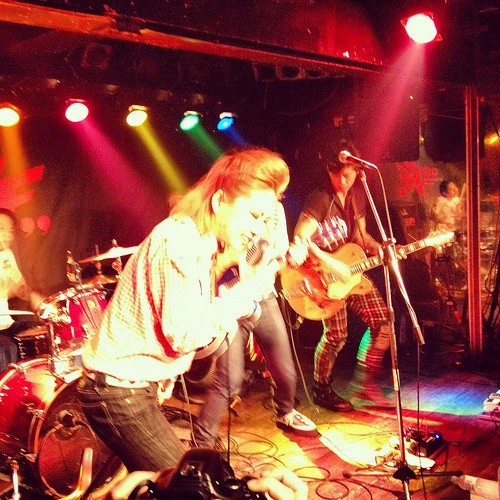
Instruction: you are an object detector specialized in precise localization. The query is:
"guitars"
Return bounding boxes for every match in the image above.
[279,228,456,321]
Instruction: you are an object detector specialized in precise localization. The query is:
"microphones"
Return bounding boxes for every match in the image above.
[58,410,86,428]
[66,251,77,282]
[245,239,269,267]
[339,151,377,170]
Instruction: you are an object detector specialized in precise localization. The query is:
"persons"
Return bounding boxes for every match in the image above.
[0,149,318,500]
[291,141,408,413]
[428,180,465,233]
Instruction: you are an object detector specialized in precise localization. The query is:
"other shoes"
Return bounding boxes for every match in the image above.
[275,408,320,438]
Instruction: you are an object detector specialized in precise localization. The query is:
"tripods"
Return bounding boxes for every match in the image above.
[342,169,463,500]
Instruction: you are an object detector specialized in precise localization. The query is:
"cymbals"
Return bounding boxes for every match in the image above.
[80,274,119,287]
[78,244,140,266]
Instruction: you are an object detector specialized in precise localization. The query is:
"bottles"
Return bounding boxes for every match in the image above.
[451,475,499,499]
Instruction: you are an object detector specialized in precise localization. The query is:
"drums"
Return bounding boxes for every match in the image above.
[14,324,52,356]
[37,286,108,348]
[0,356,121,500]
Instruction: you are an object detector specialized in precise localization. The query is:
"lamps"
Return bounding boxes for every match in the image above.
[400,0,444,49]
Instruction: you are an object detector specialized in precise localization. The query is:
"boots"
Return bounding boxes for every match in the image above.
[350,381,397,409]
[310,383,353,412]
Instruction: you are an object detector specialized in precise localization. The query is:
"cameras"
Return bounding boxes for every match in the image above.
[147,448,268,500]
